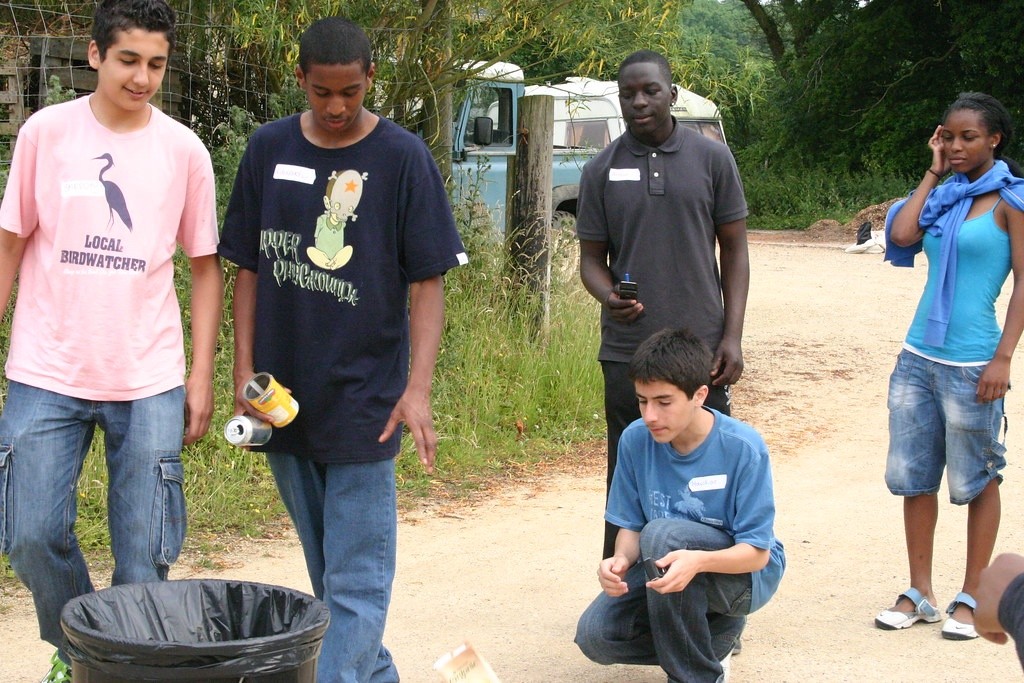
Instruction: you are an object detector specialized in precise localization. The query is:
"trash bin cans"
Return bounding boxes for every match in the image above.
[58,578,332,683]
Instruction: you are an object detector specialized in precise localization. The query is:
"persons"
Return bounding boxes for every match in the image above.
[577,49,751,561]
[574,328,785,683]
[876,91,1024,640]
[217,17,470,683]
[0,0,221,683]
[974,554,1024,672]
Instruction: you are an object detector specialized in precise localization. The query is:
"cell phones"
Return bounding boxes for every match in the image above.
[619,280,638,302]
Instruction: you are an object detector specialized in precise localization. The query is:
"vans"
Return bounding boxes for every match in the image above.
[483,75,729,148]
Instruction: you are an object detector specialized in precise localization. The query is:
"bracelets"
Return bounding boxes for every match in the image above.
[929,169,940,179]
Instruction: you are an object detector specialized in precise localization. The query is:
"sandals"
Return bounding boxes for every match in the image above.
[941,591,985,641]
[873,588,942,630]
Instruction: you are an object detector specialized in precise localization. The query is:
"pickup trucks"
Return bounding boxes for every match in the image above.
[371,55,605,299]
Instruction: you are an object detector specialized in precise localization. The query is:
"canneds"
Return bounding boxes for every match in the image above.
[224,415,273,446]
[243,372,299,428]
